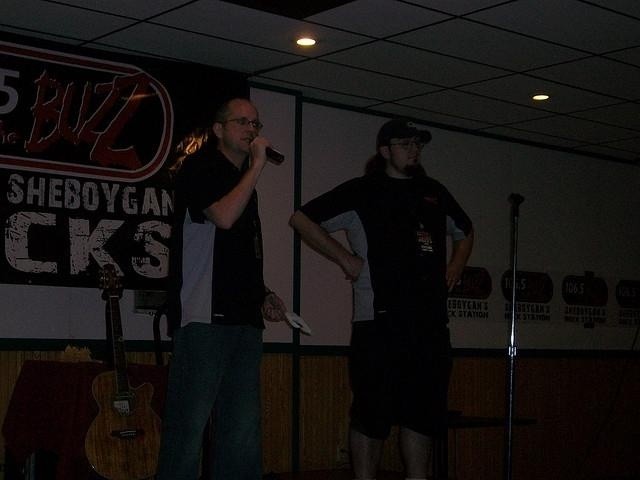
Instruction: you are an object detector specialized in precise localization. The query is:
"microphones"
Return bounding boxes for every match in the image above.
[507,192,525,205]
[248,137,285,167]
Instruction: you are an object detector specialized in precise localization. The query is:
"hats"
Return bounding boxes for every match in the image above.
[377,118,432,144]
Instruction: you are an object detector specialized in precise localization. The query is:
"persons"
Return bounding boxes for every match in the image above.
[154,94,287,480]
[288,117,474,478]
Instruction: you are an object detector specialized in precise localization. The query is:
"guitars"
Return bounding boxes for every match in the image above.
[85,263,162,479]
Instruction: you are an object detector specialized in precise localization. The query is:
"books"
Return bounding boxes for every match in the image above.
[284,309,312,336]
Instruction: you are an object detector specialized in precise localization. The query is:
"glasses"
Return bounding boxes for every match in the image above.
[225,118,263,128]
[391,142,424,152]
